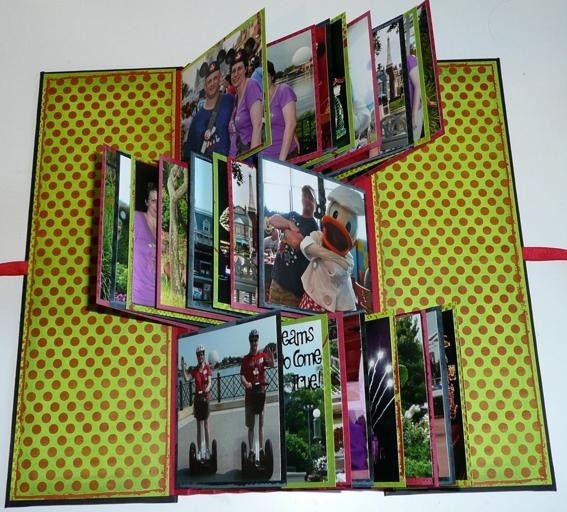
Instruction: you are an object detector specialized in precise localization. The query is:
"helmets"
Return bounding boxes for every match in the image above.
[196,345,205,352]
[249,330,259,340]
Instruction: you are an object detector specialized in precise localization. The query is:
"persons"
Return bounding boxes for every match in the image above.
[180,34,435,163]
[132,185,160,306]
[267,184,321,308]
[240,327,275,466]
[263,228,282,252]
[177,344,212,462]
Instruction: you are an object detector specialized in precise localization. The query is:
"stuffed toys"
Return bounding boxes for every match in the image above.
[297,186,364,313]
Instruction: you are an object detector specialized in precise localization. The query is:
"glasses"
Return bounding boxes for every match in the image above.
[148,199,157,202]
[251,340,257,342]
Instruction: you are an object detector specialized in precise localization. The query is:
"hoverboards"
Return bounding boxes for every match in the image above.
[242,382,273,482]
[189,389,218,476]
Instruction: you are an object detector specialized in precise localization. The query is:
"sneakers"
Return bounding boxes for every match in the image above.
[248,450,265,465]
[197,448,210,461]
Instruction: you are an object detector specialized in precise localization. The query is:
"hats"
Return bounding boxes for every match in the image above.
[327,186,365,216]
[302,185,316,200]
